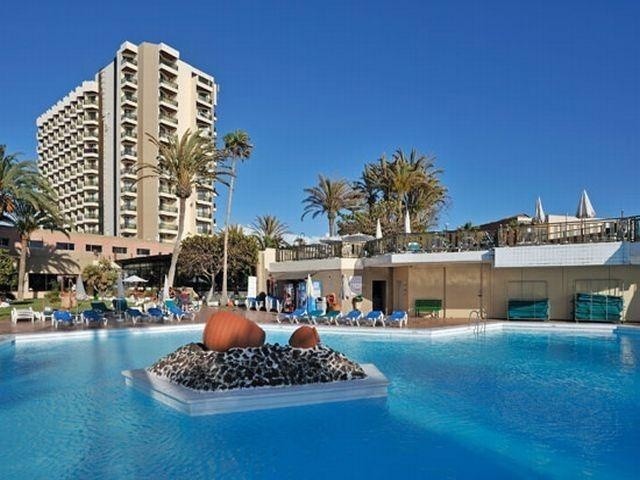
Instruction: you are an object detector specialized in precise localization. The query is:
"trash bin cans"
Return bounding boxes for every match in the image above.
[316,296,327,315]
[60,291,71,309]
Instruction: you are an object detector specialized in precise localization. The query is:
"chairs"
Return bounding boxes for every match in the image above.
[276,308,408,329]
[8,292,197,327]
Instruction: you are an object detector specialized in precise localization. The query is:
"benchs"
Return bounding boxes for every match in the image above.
[414,300,443,319]
